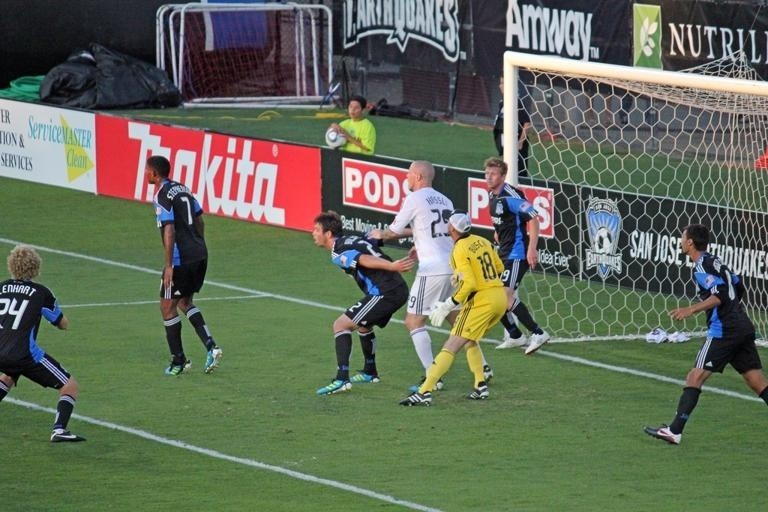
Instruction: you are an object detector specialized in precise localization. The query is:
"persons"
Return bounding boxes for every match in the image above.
[0,243,88,443]
[312,209,417,396]
[144,155,222,376]
[483,156,551,355]
[331,95,376,156]
[494,70,531,177]
[366,158,494,393]
[399,209,509,406]
[643,219,767,444]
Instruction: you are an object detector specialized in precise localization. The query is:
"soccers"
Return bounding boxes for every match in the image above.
[325,128,347,149]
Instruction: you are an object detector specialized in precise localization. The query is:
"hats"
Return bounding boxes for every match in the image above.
[449,213,472,233]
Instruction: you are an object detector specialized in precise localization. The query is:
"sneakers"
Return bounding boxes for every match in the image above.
[50,427,88,443]
[351,372,381,383]
[642,425,682,445]
[401,363,493,405]
[316,379,352,395]
[205,347,223,373]
[165,358,192,376]
[495,333,526,350]
[524,329,551,355]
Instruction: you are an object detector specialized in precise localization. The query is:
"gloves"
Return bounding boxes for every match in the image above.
[428,295,457,328]
